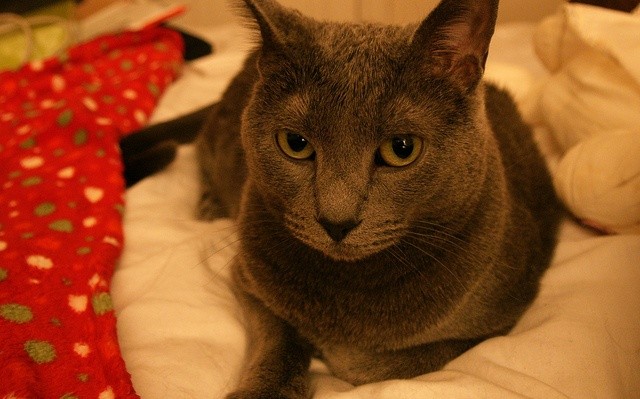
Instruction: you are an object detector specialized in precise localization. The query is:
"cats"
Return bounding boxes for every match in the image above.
[191,0,563,399]
